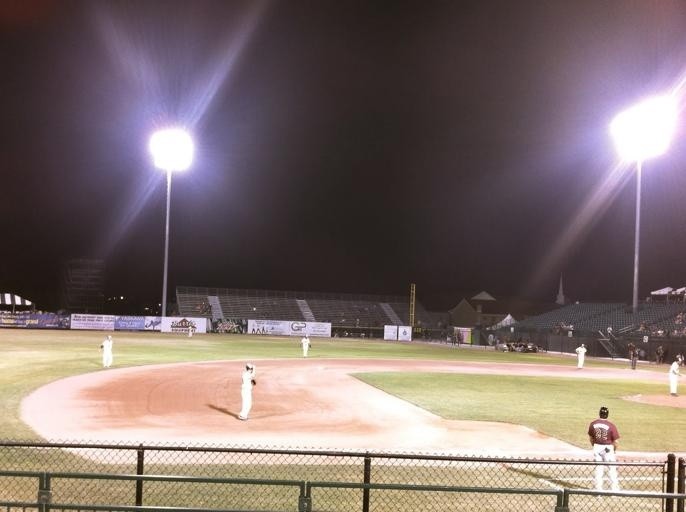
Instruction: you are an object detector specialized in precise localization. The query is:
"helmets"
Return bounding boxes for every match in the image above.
[246,364,253,371]
[600,407,608,419]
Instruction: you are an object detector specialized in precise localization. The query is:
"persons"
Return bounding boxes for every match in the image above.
[188,322,193,338]
[607,326,612,337]
[299,334,313,358]
[627,321,664,370]
[214,319,247,334]
[487,331,548,353]
[100,335,113,369]
[587,407,622,501]
[575,343,587,370]
[669,354,682,398]
[238,363,256,421]
[424,327,463,346]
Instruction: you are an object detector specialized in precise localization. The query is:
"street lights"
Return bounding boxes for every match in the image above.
[151,128,195,314]
[615,100,671,314]
